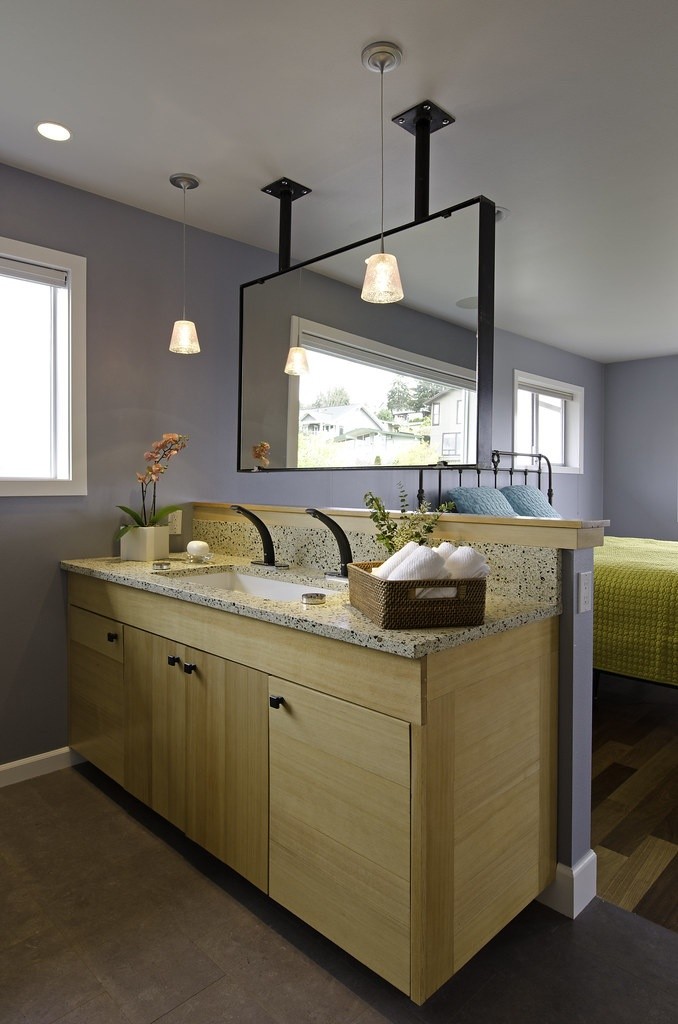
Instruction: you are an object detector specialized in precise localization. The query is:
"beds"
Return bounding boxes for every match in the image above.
[420,450,678,703]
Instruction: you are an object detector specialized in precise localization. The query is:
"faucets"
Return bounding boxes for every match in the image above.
[228,504,291,572]
[305,508,353,581]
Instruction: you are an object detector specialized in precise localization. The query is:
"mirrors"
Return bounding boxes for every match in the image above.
[237,102,498,473]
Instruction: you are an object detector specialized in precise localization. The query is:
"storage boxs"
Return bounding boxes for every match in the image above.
[348,563,487,629]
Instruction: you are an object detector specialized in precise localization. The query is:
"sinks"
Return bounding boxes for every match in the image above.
[150,564,354,603]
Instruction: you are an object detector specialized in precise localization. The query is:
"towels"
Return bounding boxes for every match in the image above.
[370,541,421,582]
[388,545,454,582]
[426,541,459,565]
[438,545,491,580]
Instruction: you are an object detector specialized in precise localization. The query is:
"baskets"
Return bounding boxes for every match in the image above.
[348,560,485,627]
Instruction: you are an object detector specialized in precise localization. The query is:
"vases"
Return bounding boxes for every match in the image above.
[120,524,169,561]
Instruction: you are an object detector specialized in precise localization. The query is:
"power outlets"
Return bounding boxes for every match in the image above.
[168,510,182,535]
[577,573,593,615]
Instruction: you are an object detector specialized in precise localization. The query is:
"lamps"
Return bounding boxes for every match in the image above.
[168,173,204,354]
[285,268,309,374]
[361,41,404,302]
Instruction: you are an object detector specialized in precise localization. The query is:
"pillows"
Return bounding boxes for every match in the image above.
[501,485,563,521]
[446,487,519,519]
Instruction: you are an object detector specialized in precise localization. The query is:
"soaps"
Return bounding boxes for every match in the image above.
[185,540,211,557]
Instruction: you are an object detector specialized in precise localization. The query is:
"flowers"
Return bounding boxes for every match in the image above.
[253,442,271,468]
[115,433,188,541]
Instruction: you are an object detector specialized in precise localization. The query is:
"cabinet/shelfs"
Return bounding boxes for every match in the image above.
[66,571,562,1005]
